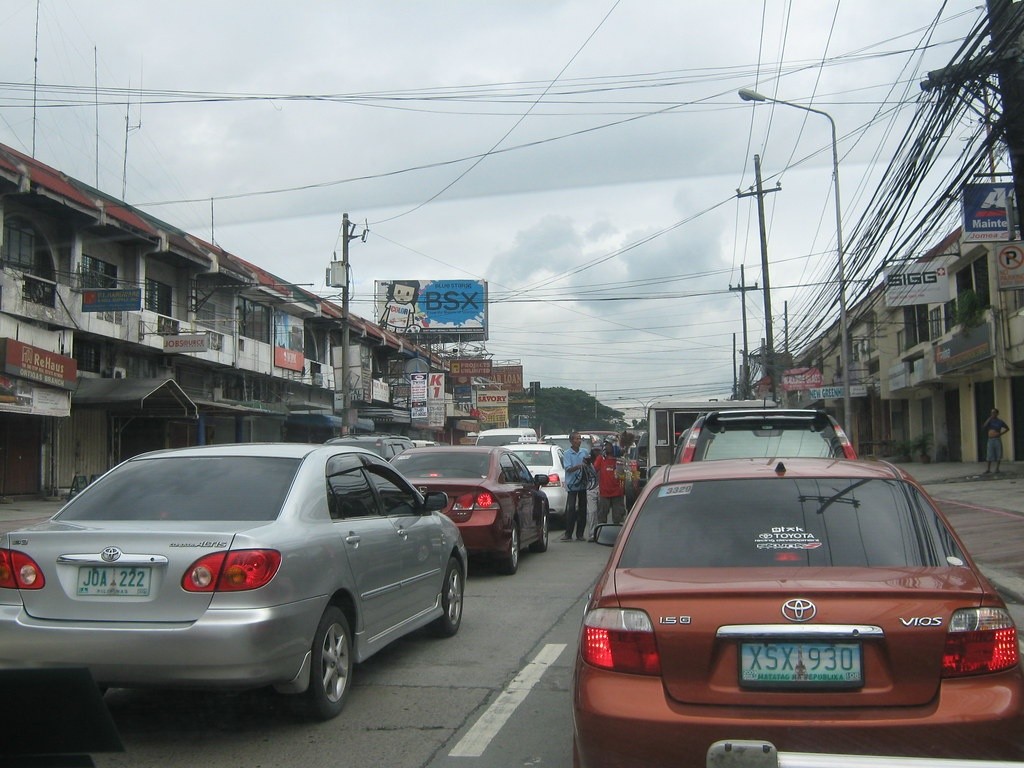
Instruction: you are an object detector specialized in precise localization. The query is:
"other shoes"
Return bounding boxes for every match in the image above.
[561,535,573,540]
[576,536,585,541]
[587,535,594,542]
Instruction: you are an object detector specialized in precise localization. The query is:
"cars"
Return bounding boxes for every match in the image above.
[1,442,467,723]
[388,445,552,575]
[539,429,621,450]
[498,443,578,521]
[410,440,439,452]
[569,457,1023,767]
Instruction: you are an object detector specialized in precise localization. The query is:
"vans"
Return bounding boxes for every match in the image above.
[324,431,416,466]
[473,425,537,448]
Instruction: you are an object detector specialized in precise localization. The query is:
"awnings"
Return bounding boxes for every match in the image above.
[72,375,199,419]
[289,412,374,432]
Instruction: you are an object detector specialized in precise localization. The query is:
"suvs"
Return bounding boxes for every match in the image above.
[666,404,857,465]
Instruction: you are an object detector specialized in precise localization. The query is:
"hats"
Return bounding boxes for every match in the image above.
[605,435,619,447]
[621,432,634,446]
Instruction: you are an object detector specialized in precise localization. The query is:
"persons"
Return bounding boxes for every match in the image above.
[586,431,635,541]
[560,432,590,541]
[984,408,1009,473]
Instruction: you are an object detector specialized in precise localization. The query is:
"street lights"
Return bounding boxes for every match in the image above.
[736,88,854,437]
[618,392,674,420]
